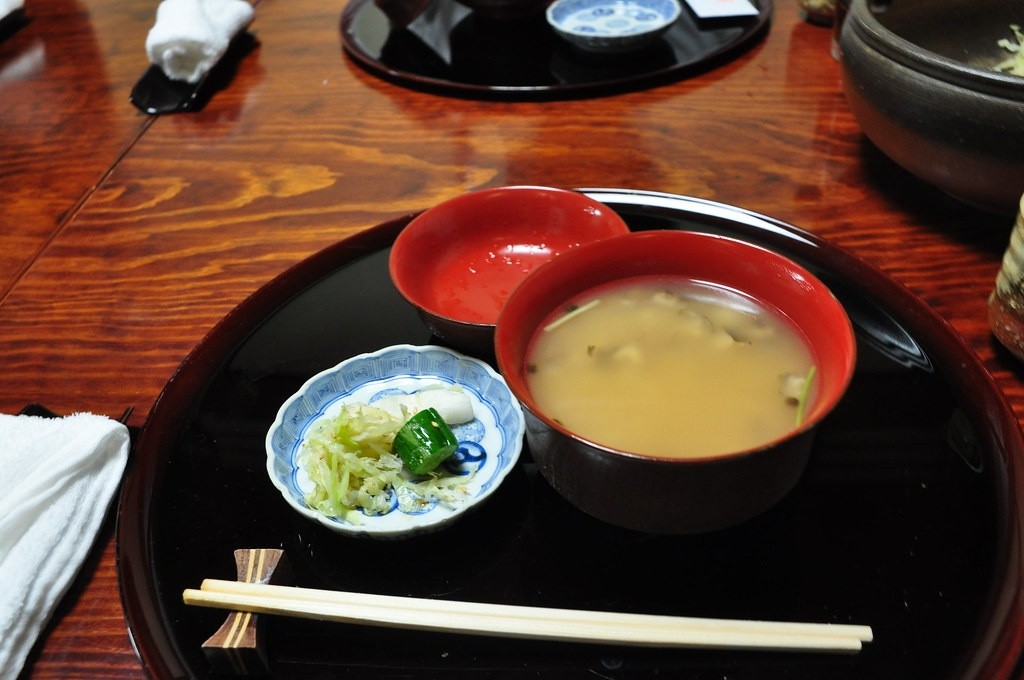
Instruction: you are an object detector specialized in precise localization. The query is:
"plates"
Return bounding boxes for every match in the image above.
[547,0,682,56]
[267,343,528,532]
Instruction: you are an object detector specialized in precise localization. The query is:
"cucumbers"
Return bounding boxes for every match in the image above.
[393,407,458,475]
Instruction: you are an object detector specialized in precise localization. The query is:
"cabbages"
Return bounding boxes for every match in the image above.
[302,401,468,516]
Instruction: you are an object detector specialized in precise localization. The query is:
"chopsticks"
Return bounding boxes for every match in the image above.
[183,579,873,649]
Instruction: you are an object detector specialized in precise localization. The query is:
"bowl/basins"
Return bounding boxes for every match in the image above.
[496,233,857,475]
[388,185,632,352]
[839,0,1024,203]
